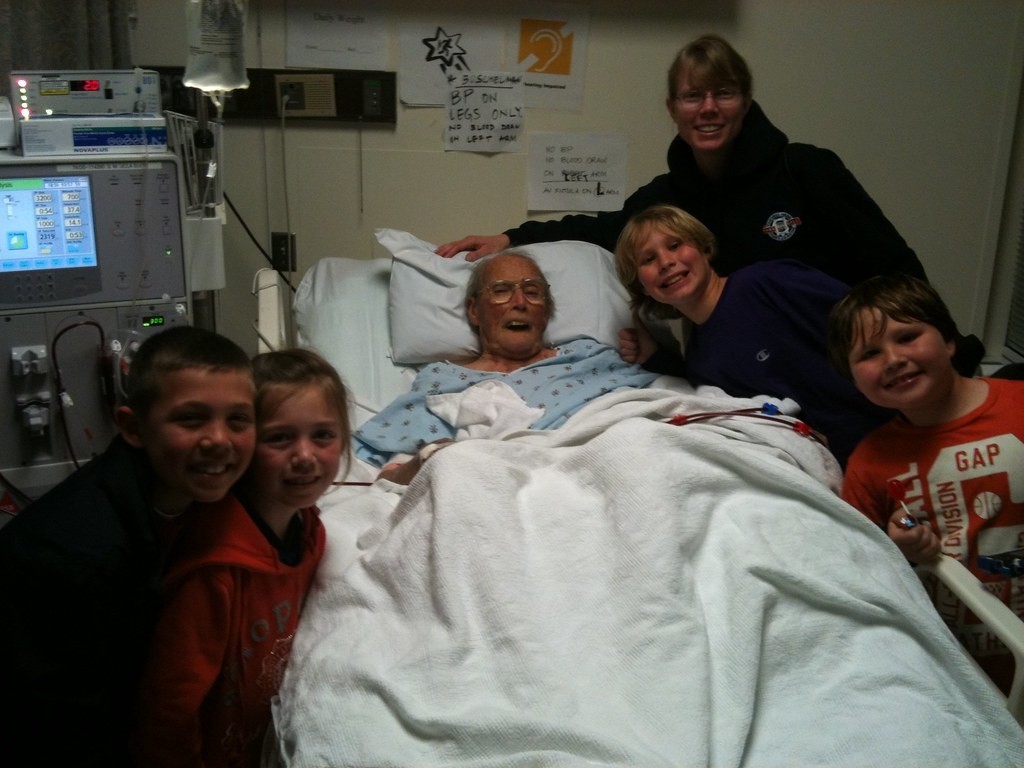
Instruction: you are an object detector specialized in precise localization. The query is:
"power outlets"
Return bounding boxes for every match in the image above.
[275,74,336,118]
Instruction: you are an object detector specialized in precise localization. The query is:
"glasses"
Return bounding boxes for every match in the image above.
[671,86,746,101]
[472,279,552,306]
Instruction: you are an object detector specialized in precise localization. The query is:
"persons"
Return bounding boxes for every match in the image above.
[435,38,986,375]
[142,351,354,767]
[834,274,1024,695]
[0,327,254,768]
[359,250,660,465]
[618,205,883,470]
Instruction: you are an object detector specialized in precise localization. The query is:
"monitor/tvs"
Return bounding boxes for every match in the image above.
[0,175,98,272]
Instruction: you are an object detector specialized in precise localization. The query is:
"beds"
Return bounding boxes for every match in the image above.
[258,257,1024,768]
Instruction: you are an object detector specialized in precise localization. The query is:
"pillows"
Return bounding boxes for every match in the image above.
[373,227,634,365]
